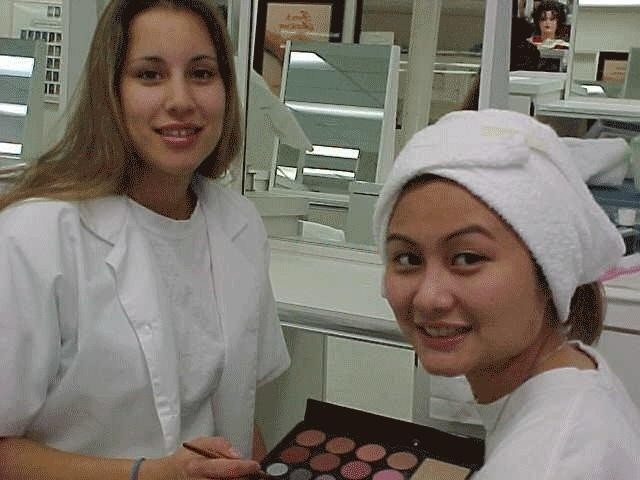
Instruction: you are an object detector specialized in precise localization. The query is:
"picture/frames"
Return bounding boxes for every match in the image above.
[249,0,364,98]
[586,51,630,128]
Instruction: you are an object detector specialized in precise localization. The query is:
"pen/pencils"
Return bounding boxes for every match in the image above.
[183,443,269,478]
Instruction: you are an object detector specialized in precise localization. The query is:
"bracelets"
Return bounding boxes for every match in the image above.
[131,455,145,479]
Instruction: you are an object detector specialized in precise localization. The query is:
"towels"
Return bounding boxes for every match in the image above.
[370,109,635,324]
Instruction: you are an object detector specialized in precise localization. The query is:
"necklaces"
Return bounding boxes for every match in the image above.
[522,336,570,370]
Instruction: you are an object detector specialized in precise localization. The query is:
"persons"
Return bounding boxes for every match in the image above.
[371,108,638,480]
[0,0,294,480]
[529,1,571,48]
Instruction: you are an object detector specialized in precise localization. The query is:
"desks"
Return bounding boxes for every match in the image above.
[257,252,639,453]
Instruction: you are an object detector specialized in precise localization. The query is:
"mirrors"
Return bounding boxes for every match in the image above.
[0,2,71,170]
[238,0,511,265]
[269,40,402,204]
[511,1,580,101]
